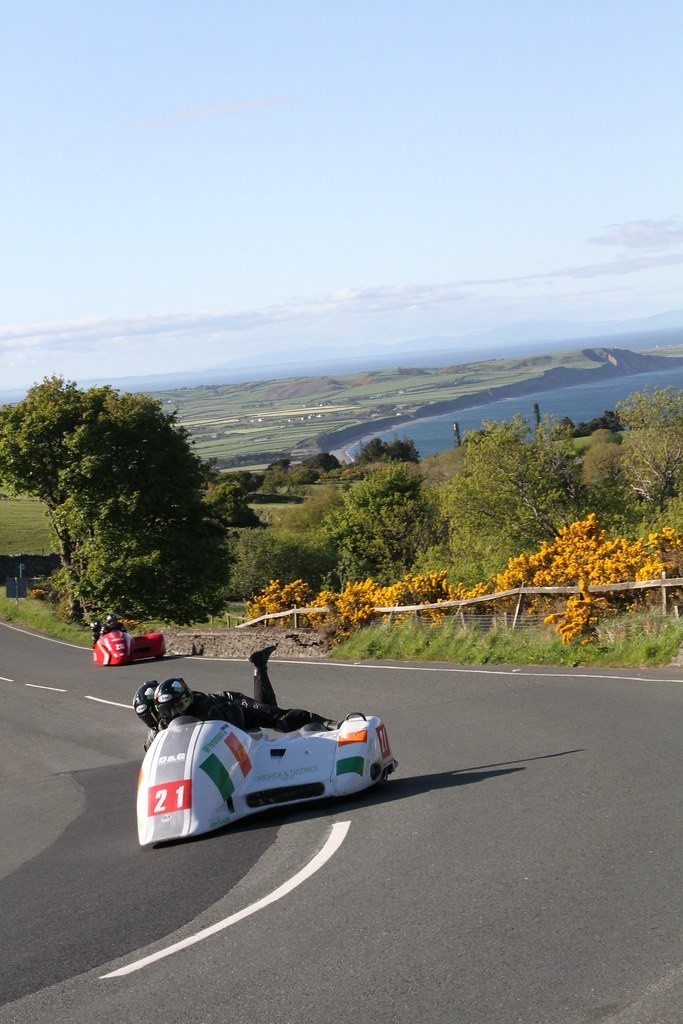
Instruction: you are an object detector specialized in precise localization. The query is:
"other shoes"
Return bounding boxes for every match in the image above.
[248,646,277,667]
[323,720,344,729]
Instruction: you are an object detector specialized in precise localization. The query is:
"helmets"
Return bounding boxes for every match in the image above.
[90,621,101,633]
[106,615,118,627]
[133,679,161,728]
[153,678,194,720]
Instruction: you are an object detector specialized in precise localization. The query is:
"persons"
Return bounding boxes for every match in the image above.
[133,645,343,752]
[90,614,127,649]
